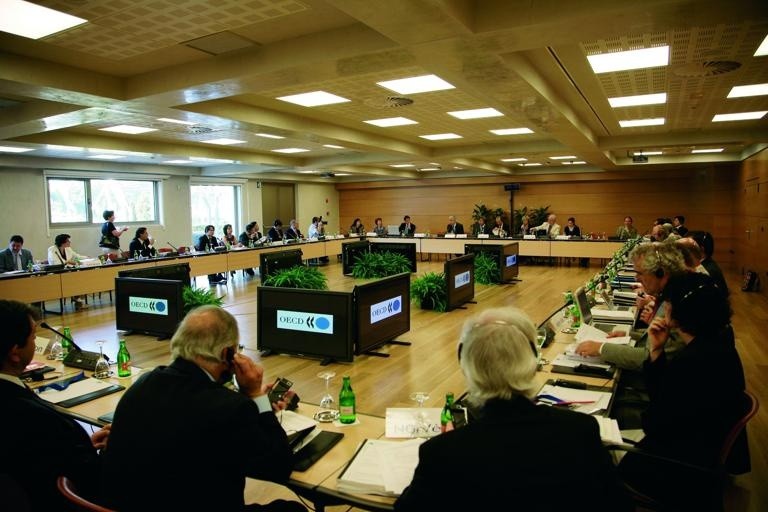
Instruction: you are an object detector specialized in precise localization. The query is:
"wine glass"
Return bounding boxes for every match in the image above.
[139,250,143,260]
[230,241,234,250]
[106,253,112,264]
[95,340,111,378]
[209,243,214,251]
[410,392,431,439]
[48,326,62,360]
[534,329,547,365]
[316,371,338,421]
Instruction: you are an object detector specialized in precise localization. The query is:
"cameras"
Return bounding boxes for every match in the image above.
[315,217,328,226]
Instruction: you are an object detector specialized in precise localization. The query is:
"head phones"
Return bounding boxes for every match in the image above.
[671,279,718,323]
[653,247,663,280]
[456,316,537,365]
[226,347,234,366]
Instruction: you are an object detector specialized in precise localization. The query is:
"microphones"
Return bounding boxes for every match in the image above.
[55,251,65,268]
[41,321,109,371]
[214,237,227,251]
[585,275,610,306]
[536,300,573,348]
[606,229,648,290]
[164,242,180,257]
[110,243,129,262]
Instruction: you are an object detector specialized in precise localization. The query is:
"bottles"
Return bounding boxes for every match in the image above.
[339,377,356,423]
[439,393,455,433]
[117,339,131,376]
[564,237,637,328]
[73,256,80,267]
[133,250,138,260]
[247,234,302,248]
[225,241,230,250]
[204,242,209,252]
[62,328,73,358]
[100,255,105,265]
[28,260,33,273]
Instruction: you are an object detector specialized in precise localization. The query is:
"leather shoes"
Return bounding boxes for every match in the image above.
[73,296,82,303]
[75,304,89,308]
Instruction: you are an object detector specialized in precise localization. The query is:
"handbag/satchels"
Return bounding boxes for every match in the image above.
[99,234,119,249]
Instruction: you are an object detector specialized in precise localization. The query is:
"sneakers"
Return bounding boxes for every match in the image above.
[453,225,454,228]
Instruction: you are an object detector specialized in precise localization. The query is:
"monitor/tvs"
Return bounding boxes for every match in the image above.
[444,253,477,310]
[114,277,184,339]
[501,242,523,285]
[258,286,354,367]
[354,273,413,358]
[342,240,370,277]
[370,242,418,275]
[258,248,303,287]
[464,244,503,279]
[119,261,191,290]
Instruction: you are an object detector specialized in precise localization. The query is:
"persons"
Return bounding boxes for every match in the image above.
[351,215,416,238]
[576,215,729,372]
[105,304,309,511]
[98,211,155,257]
[198,216,330,281]
[392,308,621,511]
[0,300,113,511]
[445,213,580,261]
[0,234,88,306]
[615,270,754,512]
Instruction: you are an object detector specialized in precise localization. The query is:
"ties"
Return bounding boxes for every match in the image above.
[16,253,23,270]
[548,225,552,234]
[499,224,501,228]
[407,224,408,228]
